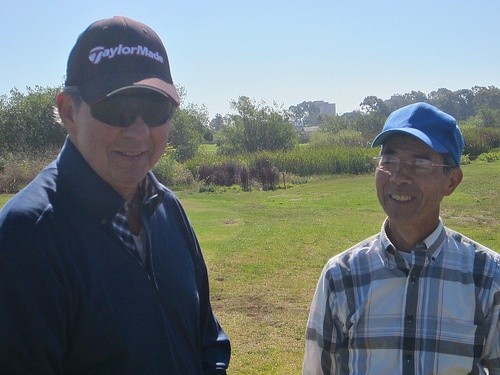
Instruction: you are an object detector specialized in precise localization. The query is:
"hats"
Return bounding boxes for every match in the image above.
[370,100,464,168]
[65,15,181,106]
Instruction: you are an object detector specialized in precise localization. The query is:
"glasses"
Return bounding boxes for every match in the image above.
[374,155,462,176]
[88,101,176,130]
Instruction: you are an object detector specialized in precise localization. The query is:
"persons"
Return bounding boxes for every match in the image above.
[0,16,231,375]
[302,102,500,375]
